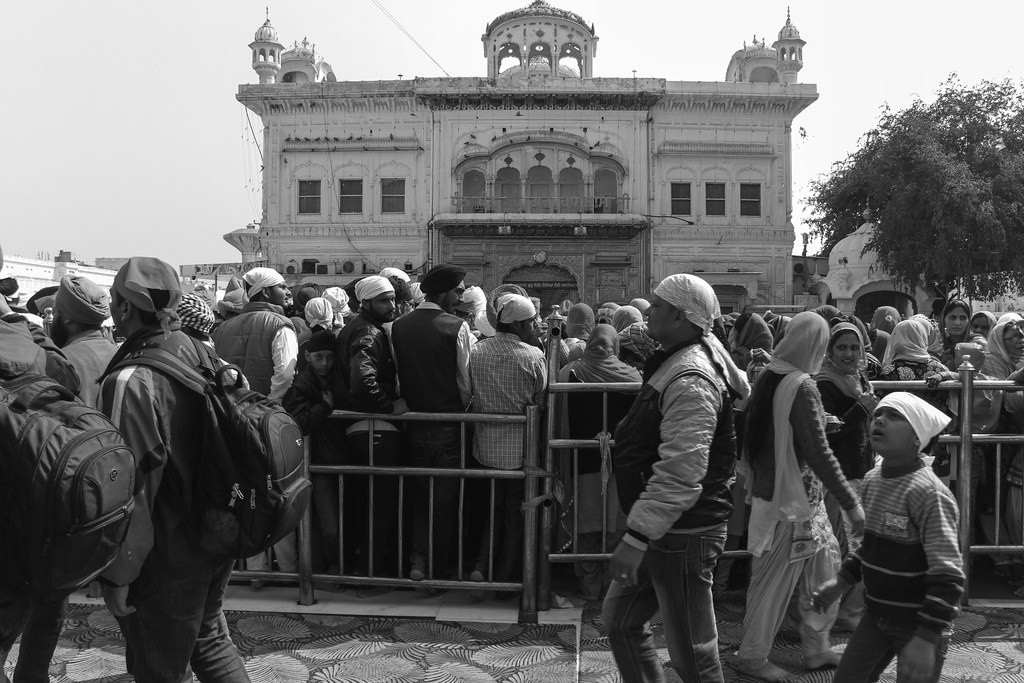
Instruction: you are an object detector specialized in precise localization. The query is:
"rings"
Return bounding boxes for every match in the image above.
[620,574,627,577]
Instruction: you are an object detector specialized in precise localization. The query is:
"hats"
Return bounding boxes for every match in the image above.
[293,282,323,312]
[58,274,112,325]
[305,331,339,353]
[176,294,215,332]
[420,263,467,294]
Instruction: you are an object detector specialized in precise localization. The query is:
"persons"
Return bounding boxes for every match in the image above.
[0,257,1024,683]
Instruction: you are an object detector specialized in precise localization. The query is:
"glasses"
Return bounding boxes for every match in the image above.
[1004,336,1024,342]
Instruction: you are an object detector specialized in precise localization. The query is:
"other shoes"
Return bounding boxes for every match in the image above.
[411,567,438,594]
[470,570,484,602]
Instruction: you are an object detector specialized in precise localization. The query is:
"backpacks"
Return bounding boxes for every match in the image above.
[100,334,314,560]
[0,347,138,601]
